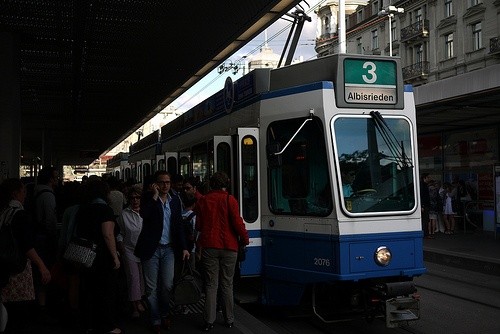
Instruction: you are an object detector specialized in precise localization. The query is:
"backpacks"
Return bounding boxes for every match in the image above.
[182,212,196,253]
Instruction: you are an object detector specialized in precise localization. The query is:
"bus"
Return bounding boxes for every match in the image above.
[106,10,428,326]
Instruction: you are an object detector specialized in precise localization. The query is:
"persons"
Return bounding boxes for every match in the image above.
[340,157,358,198]
[420,173,472,240]
[0,166,203,334]
[195,171,249,334]
[133,170,191,326]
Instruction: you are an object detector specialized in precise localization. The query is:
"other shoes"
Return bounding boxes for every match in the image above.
[153,325,160,332]
[137,304,146,312]
[208,321,216,328]
[225,322,233,327]
[132,311,140,318]
[164,319,173,328]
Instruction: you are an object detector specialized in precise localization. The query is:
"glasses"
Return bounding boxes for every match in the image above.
[182,186,194,191]
[345,173,357,176]
[129,196,141,200]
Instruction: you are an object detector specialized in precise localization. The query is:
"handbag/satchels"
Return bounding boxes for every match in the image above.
[174,256,202,305]
[64,242,96,268]
[0,206,27,273]
